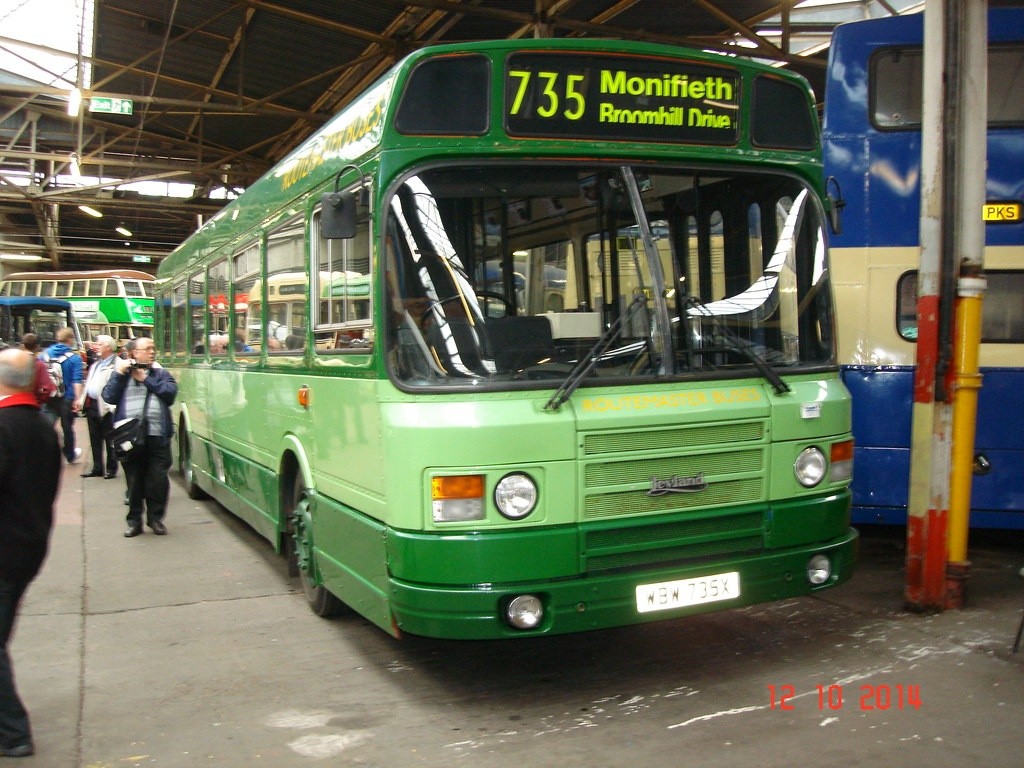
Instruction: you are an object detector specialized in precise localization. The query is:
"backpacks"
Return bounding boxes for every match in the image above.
[42,352,74,398]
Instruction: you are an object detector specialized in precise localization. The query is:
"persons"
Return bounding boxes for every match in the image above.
[0,326,179,768]
[209,330,280,354]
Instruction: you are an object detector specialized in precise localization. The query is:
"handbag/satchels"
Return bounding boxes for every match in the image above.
[109,419,147,462]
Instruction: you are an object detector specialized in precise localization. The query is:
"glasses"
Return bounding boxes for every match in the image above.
[134,346,155,351]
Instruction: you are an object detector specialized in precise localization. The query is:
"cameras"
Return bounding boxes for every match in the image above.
[129,362,148,371]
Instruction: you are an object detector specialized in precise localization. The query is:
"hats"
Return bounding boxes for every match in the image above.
[0,338,10,348]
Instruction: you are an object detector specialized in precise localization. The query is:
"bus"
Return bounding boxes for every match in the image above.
[157,266,370,356]
[153,38,861,642]
[1,295,88,379]
[564,5,1024,536]
[0,268,156,351]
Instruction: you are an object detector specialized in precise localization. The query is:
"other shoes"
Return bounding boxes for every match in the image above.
[147,520,166,534]
[0,743,33,756]
[67,447,82,464]
[81,471,102,476]
[124,525,142,537]
[125,490,130,504]
[104,472,114,478]
